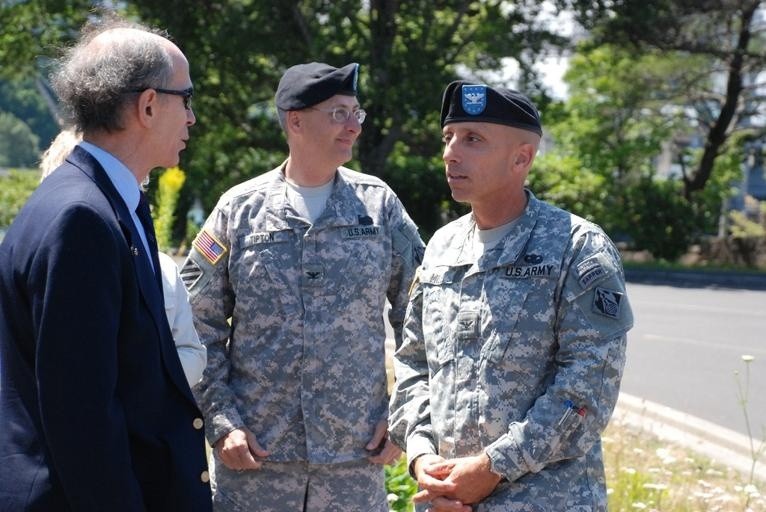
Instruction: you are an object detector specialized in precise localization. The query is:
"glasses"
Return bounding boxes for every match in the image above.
[121,86,194,109]
[304,107,367,124]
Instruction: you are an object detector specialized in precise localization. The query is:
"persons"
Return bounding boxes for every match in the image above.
[0,15,214,510]
[176,57,430,512]
[35,128,210,392]
[383,79,635,511]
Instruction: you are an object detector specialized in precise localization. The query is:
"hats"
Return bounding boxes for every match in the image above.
[273,63,359,110]
[441,80,529,130]
